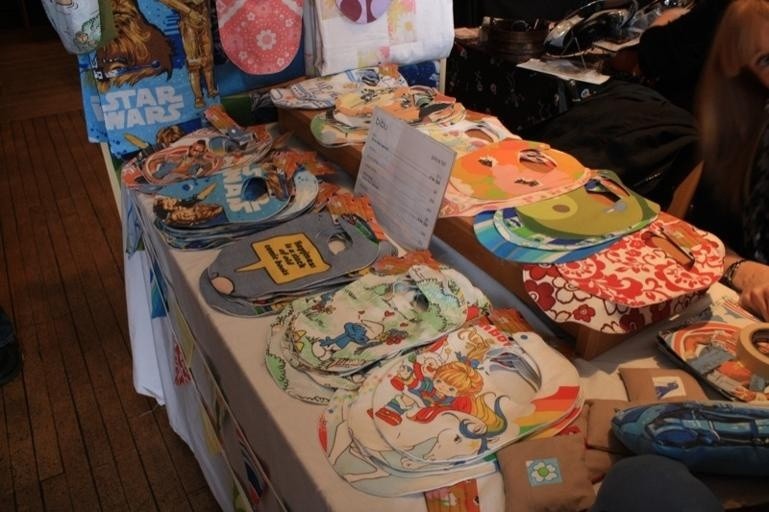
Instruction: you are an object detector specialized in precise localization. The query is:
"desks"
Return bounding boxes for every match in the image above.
[103,66,769,512]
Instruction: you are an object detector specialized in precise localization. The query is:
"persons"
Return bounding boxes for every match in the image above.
[159,0,219,108]
[698,1,769,322]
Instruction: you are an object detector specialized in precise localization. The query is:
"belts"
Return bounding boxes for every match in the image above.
[629,0,695,30]
[478,18,548,62]
[543,0,640,55]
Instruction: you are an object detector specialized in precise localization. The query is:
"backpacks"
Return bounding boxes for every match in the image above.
[526,80,699,196]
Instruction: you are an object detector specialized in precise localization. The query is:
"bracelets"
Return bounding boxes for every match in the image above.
[721,258,750,287]
